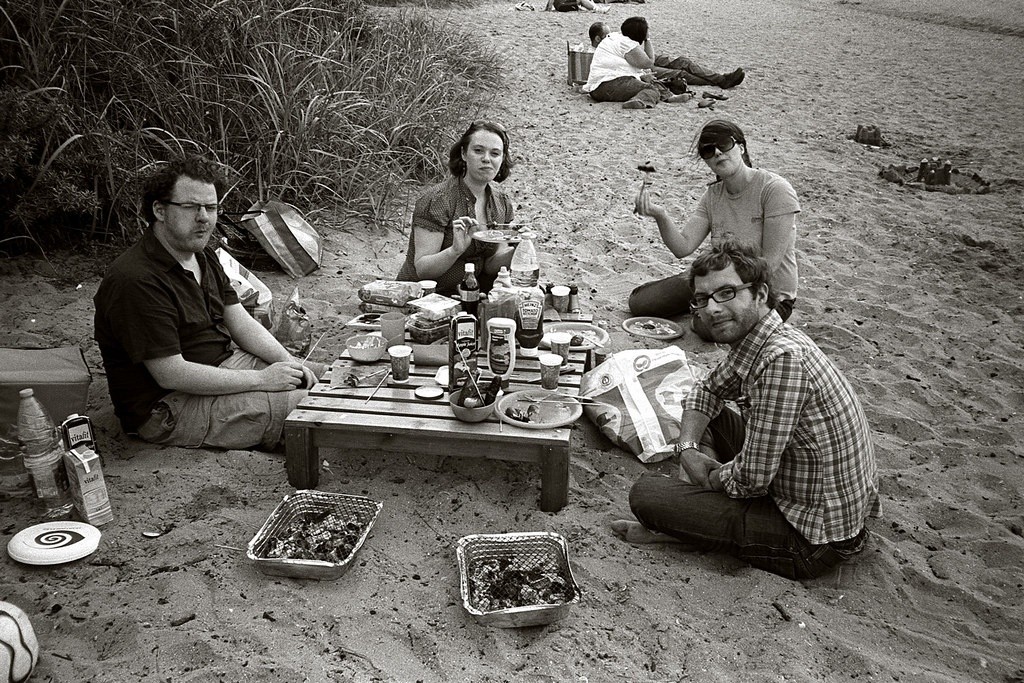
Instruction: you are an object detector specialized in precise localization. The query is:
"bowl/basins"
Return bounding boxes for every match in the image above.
[345,334,388,361]
[449,389,497,422]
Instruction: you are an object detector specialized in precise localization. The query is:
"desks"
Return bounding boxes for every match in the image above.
[284,314,594,511]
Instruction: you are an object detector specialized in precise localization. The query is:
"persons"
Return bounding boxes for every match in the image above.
[629,120,801,340]
[92,158,331,448]
[583,16,660,109]
[588,22,745,90]
[396,119,539,301]
[611,242,878,581]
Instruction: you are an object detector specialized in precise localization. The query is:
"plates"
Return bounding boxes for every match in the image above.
[345,312,383,330]
[493,390,583,430]
[621,317,685,340]
[471,229,538,243]
[541,322,610,349]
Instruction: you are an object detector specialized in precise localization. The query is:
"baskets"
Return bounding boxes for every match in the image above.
[456,532,581,628]
[247,492,383,580]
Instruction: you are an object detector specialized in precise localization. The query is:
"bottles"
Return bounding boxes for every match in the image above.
[514,287,545,356]
[17,388,73,518]
[460,263,479,330]
[493,266,511,288]
[510,232,539,287]
[487,318,517,390]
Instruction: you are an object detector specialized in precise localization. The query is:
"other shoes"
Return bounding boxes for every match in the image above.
[722,73,745,89]
[698,97,716,108]
[724,68,742,79]
[596,5,611,14]
[702,91,729,100]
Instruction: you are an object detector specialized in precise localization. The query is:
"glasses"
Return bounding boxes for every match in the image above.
[167,202,224,217]
[690,283,752,308]
[472,120,508,134]
[700,136,737,160]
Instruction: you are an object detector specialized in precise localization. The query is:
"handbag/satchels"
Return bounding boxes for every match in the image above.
[579,345,696,464]
[222,201,323,277]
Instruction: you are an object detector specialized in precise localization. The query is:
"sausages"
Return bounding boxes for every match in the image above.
[637,164,657,172]
[457,370,501,407]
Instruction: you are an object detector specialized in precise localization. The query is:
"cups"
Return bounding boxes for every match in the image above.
[380,312,406,352]
[551,286,571,313]
[418,280,437,297]
[388,345,412,383]
[550,332,571,368]
[539,354,563,391]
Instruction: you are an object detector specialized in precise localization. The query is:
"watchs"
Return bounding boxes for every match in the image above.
[674,441,700,455]
